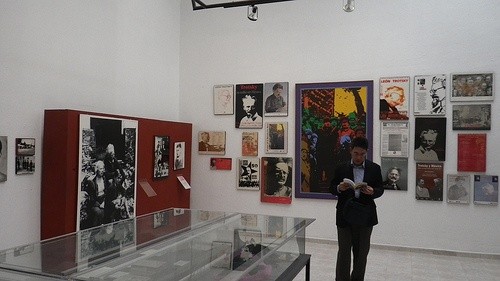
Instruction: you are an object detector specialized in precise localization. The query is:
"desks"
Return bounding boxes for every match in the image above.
[0,207,316,281]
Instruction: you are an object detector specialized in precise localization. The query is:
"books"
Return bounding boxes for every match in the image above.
[343,178,367,190]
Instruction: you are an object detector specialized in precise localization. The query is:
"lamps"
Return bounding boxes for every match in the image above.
[246,3,259,22]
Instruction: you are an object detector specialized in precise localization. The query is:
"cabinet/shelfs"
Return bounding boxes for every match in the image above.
[40,109,192,277]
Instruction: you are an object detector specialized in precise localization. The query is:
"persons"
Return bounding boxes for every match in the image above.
[80,138,183,247]
[328,137,384,281]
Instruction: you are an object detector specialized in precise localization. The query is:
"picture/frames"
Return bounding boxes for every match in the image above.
[294,80,374,200]
